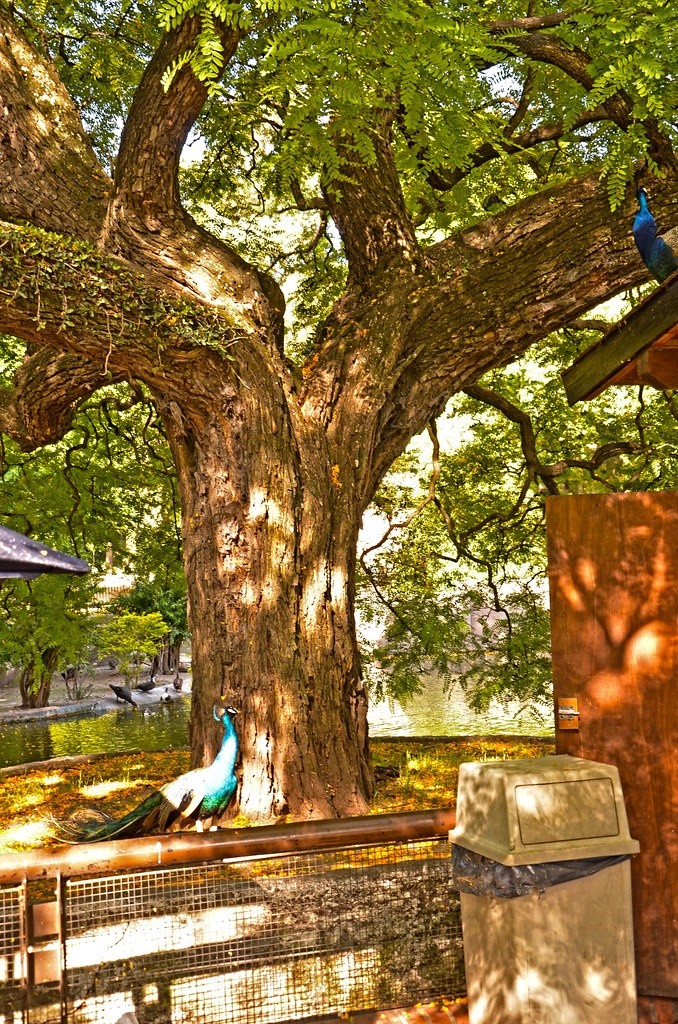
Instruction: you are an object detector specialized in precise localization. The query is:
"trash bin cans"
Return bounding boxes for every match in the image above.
[447,753,640,1024]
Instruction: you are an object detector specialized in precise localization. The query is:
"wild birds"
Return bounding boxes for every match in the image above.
[109,671,184,709]
[36,704,244,846]
